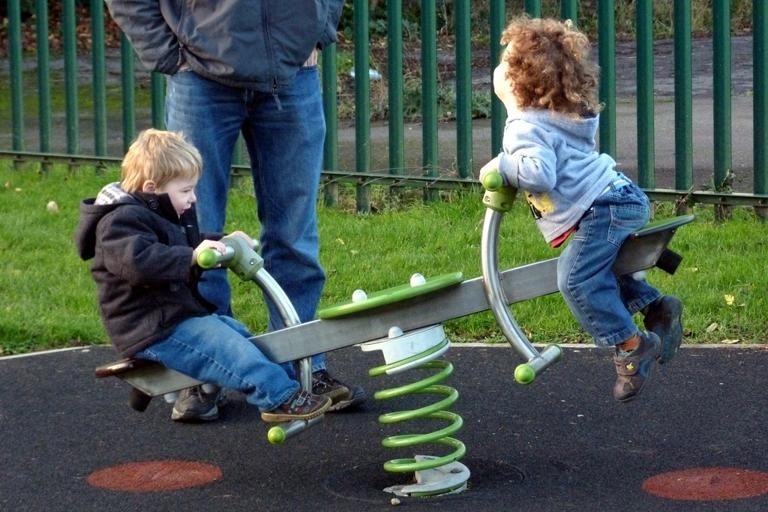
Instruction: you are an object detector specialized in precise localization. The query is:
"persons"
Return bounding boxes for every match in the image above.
[75,128,350,421]
[478,12,683,404]
[105,0,366,421]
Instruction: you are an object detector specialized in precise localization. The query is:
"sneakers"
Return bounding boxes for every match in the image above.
[610,329,664,404]
[642,295,684,364]
[169,380,226,424]
[258,387,336,424]
[306,370,349,402]
[324,371,369,412]
